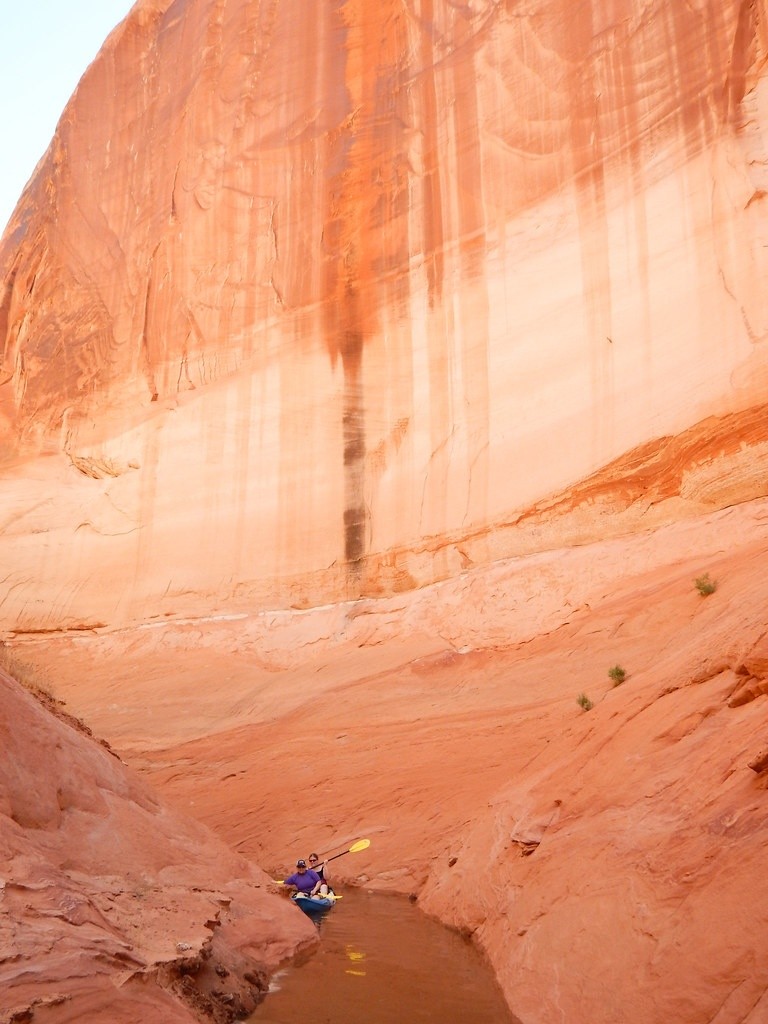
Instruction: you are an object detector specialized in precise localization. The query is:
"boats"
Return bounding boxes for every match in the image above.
[293,891,339,912]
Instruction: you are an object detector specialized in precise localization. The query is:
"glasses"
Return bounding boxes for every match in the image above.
[309,860,316,862]
[297,865,305,868]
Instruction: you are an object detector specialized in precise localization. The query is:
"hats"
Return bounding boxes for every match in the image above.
[296,860,306,865]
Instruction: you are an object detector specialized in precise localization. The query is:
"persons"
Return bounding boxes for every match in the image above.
[307,853,331,895]
[276,859,322,900]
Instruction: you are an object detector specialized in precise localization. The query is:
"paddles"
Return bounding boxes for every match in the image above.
[272,839,371,886]
[293,889,343,899]
